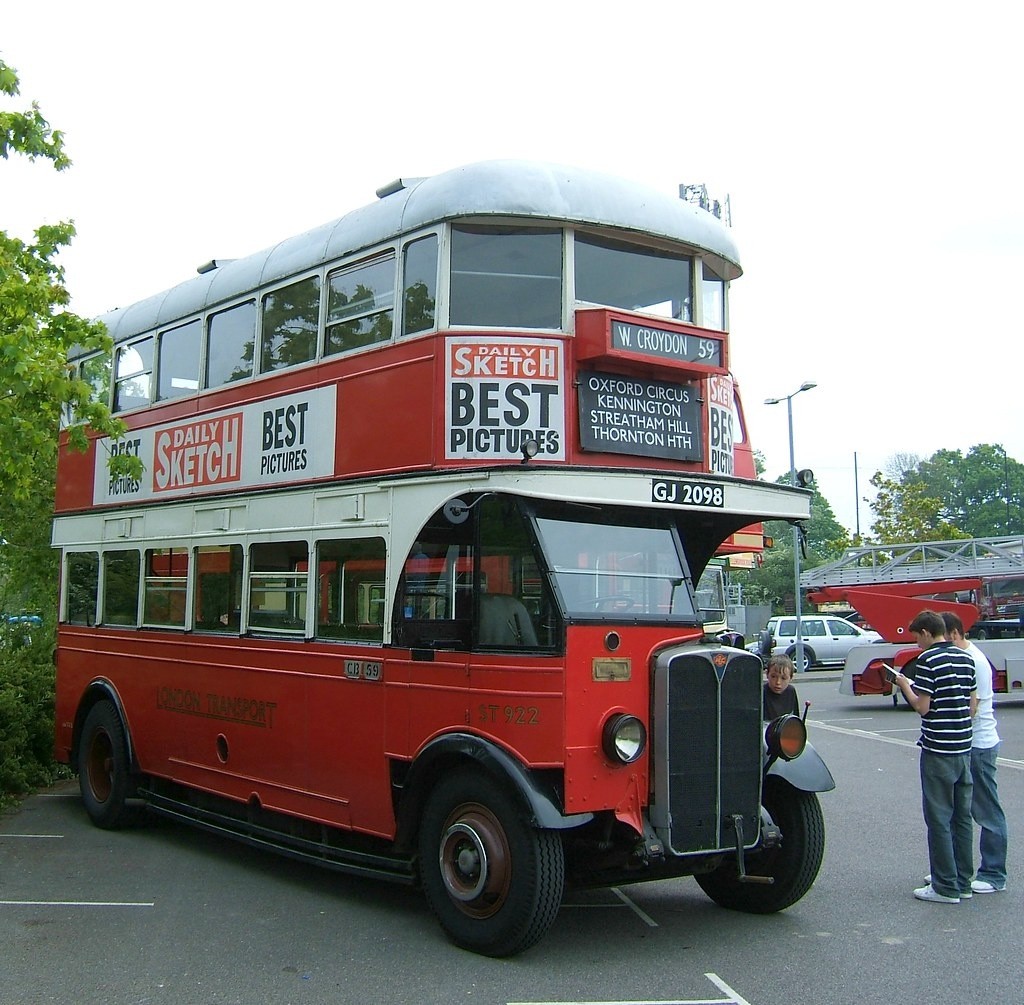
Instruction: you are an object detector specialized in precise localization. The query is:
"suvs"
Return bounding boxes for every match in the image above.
[758,615,887,672]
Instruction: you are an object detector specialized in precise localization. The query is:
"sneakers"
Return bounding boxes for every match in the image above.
[914,885,960,904]
[959,892,973,898]
[924,874,931,884]
[971,880,1006,893]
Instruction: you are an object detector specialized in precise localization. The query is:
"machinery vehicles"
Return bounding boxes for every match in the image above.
[798,533,1024,708]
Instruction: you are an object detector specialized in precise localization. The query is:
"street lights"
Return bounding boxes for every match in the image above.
[762,383,819,675]
[995,449,1010,536]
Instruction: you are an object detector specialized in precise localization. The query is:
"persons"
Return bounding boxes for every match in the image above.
[940,612,1006,893]
[895,611,977,902]
[763,654,799,722]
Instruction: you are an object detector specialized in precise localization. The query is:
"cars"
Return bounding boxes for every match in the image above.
[930,588,977,605]
[745,640,761,660]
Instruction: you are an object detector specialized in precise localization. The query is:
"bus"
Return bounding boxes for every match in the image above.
[596,550,729,640]
[48,157,838,961]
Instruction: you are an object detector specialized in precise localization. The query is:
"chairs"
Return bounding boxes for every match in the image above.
[474,592,539,649]
